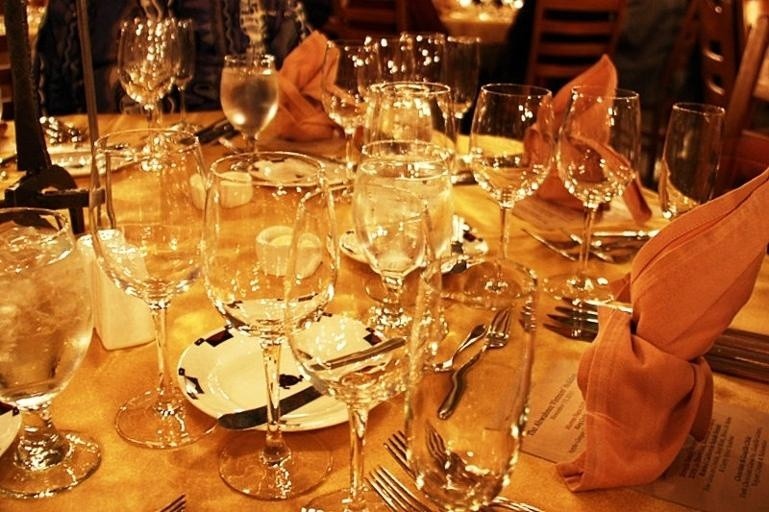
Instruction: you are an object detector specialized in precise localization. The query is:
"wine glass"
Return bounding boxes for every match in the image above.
[0,0,724,512]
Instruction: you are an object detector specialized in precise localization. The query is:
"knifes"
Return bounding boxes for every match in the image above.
[543,296,769,385]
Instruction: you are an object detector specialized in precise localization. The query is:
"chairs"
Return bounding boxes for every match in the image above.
[619,0,769,204]
[512,1,632,137]
[336,1,408,86]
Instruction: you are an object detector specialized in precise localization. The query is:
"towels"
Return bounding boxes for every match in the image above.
[258,30,345,142]
[554,167,769,492]
